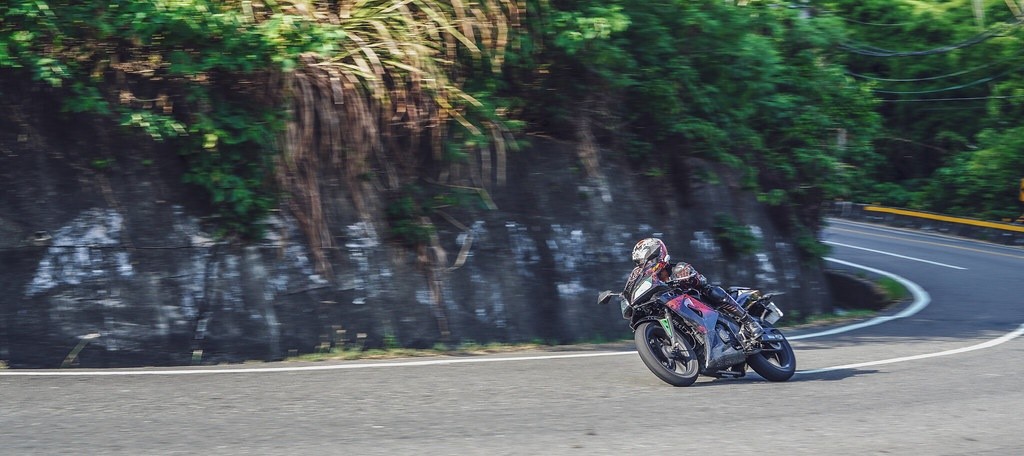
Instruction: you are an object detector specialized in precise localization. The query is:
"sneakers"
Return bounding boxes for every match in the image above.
[739,312,764,340]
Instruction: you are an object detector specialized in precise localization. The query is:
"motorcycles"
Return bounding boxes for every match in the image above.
[597,244,797,387]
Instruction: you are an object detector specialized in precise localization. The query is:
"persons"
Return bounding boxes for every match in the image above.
[625,236,765,380]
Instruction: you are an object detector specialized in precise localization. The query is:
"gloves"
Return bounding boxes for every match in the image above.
[667,278,682,288]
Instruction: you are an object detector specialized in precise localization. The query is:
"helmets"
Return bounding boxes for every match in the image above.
[632,238,670,277]
